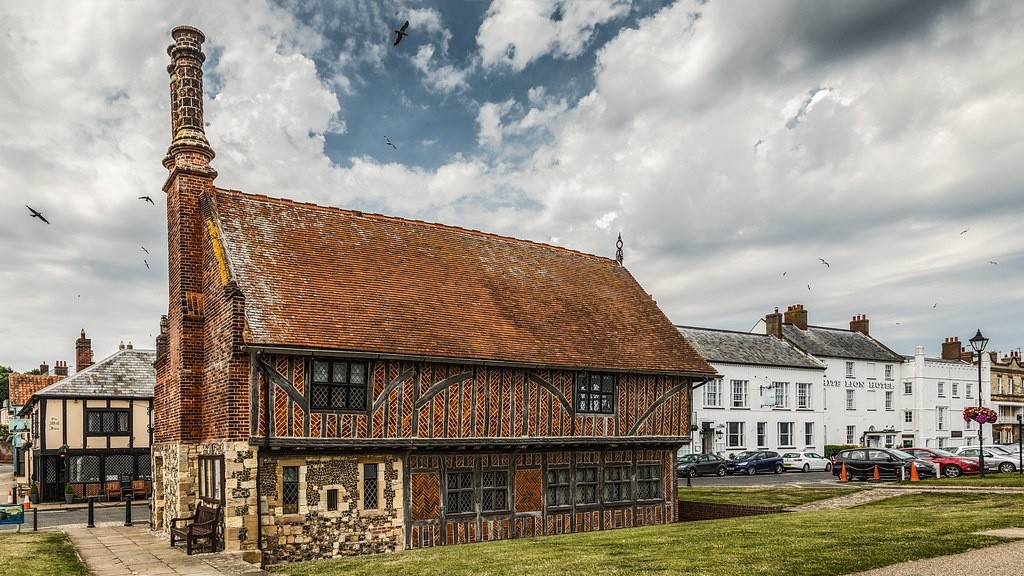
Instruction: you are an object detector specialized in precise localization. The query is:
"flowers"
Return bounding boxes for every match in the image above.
[962,406,998,424]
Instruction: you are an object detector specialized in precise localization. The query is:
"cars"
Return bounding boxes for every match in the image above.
[832,448,936,481]
[781,453,832,472]
[677,454,727,477]
[954,445,1024,473]
[726,451,784,475]
[898,447,989,478]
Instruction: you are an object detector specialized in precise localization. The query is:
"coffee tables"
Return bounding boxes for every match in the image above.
[122,485,133,501]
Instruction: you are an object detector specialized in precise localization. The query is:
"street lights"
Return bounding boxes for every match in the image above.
[1017,414,1022,474]
[969,329,989,476]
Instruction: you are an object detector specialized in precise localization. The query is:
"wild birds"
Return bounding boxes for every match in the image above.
[808,284,810,290]
[26,205,50,224]
[141,247,149,254]
[932,303,937,309]
[989,261,997,265]
[960,228,970,235]
[393,21,410,46]
[384,135,396,149]
[144,260,149,268]
[818,258,829,267]
[139,196,154,205]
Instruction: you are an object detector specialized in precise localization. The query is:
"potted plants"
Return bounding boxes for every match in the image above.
[29,483,40,504]
[64,483,74,503]
[98,489,106,502]
[74,495,84,504]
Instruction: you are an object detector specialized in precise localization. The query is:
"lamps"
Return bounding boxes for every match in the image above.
[699,429,708,439]
[717,430,723,440]
[860,436,864,442]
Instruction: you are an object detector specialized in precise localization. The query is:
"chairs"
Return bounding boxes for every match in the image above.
[106,482,122,503]
[132,480,148,501]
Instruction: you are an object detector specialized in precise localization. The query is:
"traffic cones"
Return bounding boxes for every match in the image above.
[910,462,920,481]
[24,491,33,509]
[873,465,880,479]
[836,463,848,483]
[7,491,13,503]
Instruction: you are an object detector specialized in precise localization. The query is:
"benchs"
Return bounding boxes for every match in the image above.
[17,483,31,498]
[170,501,224,555]
